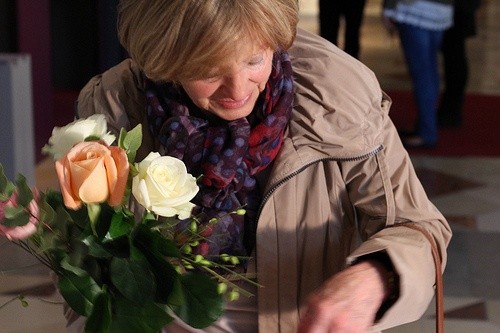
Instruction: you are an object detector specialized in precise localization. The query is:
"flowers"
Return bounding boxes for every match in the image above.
[0,114,199,333]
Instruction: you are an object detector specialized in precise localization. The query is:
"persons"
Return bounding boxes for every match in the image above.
[317,0,368,61]
[76,0,453,333]
[434,0,479,149]
[383,0,456,149]
